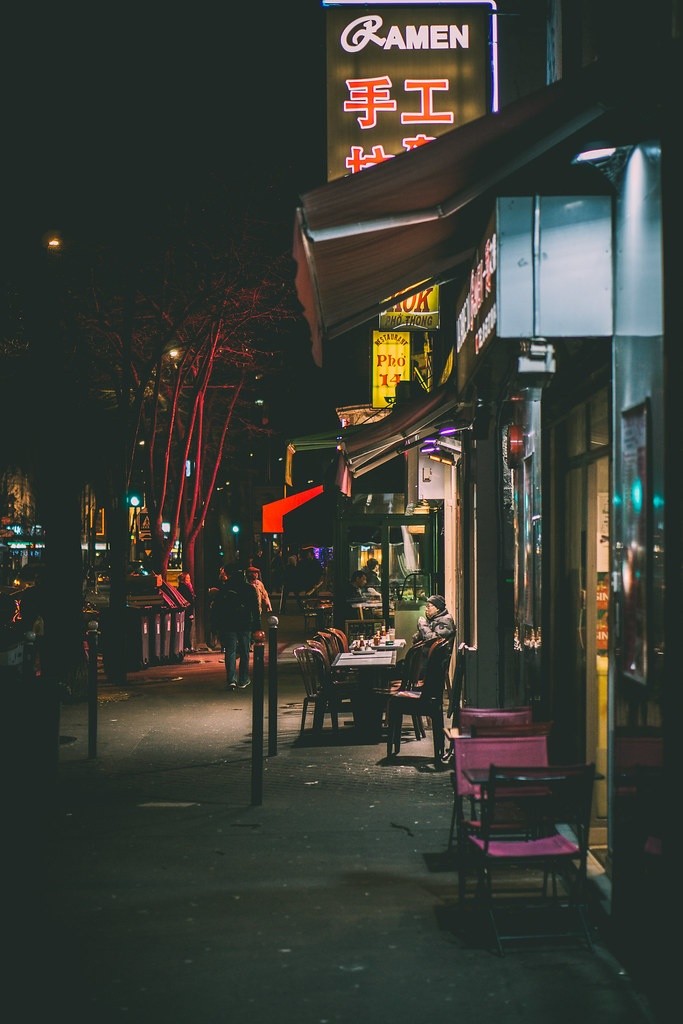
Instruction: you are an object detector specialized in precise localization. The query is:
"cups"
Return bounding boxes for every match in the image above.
[373,637,380,646]
[389,628,395,641]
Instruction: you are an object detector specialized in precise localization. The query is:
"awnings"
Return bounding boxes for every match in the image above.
[291,72,610,371]
[336,379,459,498]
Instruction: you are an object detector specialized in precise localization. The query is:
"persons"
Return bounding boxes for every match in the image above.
[232,545,306,594]
[278,547,324,615]
[387,595,456,682]
[203,567,272,663]
[176,573,195,652]
[345,571,367,620]
[209,569,262,688]
[361,558,382,585]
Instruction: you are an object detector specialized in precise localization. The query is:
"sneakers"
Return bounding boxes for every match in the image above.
[239,681,251,688]
[225,683,236,690]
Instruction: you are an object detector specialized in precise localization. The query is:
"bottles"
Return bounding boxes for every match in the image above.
[381,626,386,636]
[385,632,390,641]
[353,642,360,651]
[360,636,365,651]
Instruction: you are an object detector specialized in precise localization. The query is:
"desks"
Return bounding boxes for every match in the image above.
[348,639,407,650]
[453,764,605,924]
[443,721,556,739]
[331,649,397,733]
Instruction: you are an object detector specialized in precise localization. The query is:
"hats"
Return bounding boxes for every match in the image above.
[428,595,445,611]
[248,567,260,572]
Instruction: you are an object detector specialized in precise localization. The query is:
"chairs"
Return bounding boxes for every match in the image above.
[460,762,595,960]
[298,595,334,631]
[385,632,456,755]
[450,705,535,824]
[447,735,559,898]
[292,627,350,742]
